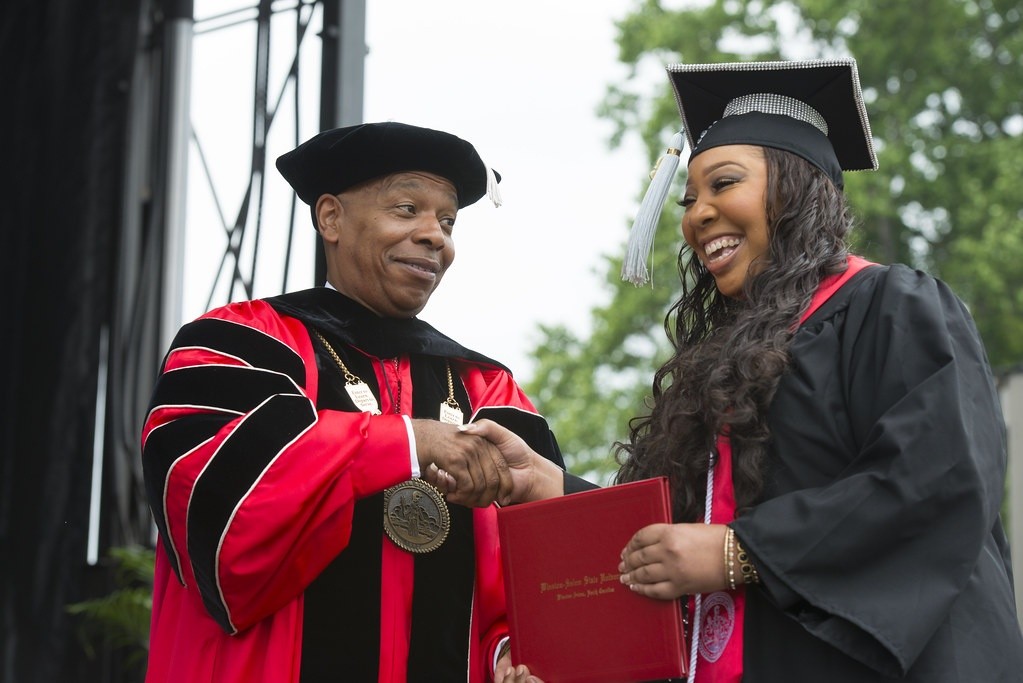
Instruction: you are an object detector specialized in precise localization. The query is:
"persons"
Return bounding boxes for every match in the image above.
[429,61,1022,682]
[140,122,569,682]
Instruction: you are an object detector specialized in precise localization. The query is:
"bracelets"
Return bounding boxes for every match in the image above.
[737,543,760,585]
[724,528,735,590]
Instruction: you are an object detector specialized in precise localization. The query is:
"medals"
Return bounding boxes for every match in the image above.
[382,478,452,554]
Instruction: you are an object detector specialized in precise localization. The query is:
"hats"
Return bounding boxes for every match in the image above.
[276,122,503,208]
[620,60,879,290]
